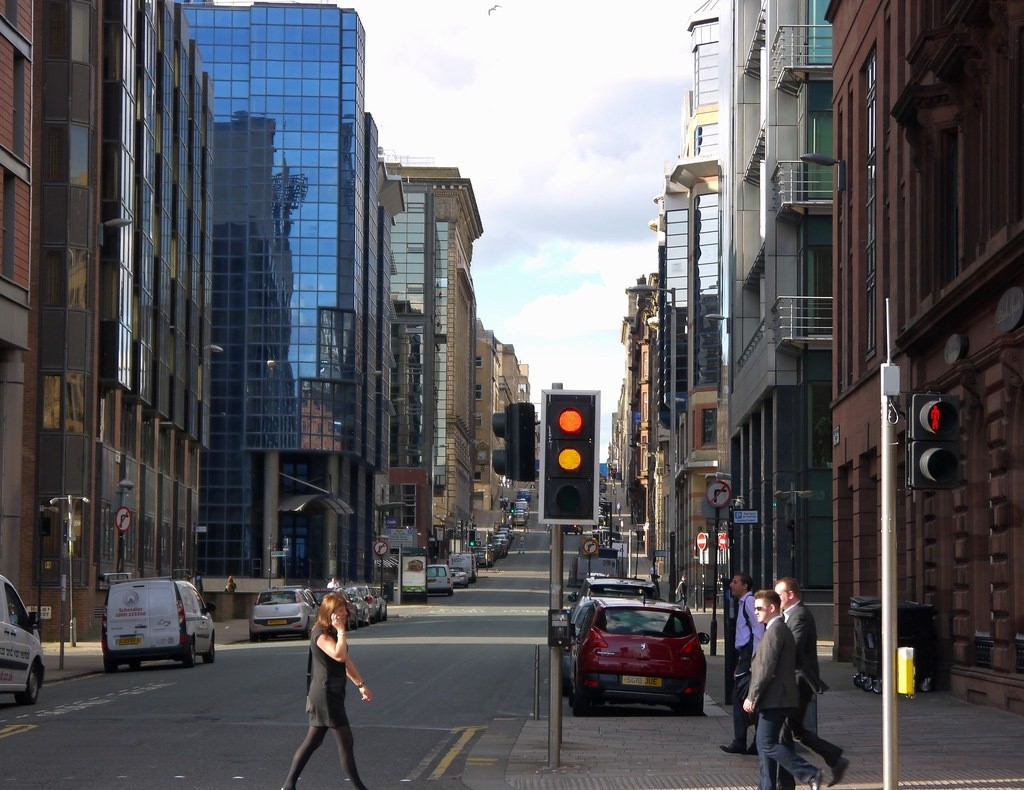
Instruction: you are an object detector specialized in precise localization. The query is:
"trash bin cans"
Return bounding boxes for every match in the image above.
[383,581,394,602]
[849,594,936,694]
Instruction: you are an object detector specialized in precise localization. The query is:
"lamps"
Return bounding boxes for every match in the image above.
[38,503,59,514]
[362,370,384,377]
[115,479,133,492]
[205,342,223,355]
[387,398,407,403]
[734,494,749,508]
[706,310,725,324]
[266,359,275,369]
[704,471,734,483]
[98,215,135,248]
[791,486,818,500]
[796,150,836,167]
[773,489,789,502]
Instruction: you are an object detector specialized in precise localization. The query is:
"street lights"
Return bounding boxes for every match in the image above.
[381,535,390,596]
[49,494,91,646]
[775,490,815,580]
[627,284,677,603]
[704,471,732,655]
[113,480,135,580]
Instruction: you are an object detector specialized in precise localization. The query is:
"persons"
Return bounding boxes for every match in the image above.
[327,577,339,589]
[675,575,688,606]
[518,534,525,554]
[617,502,624,516]
[224,576,237,593]
[774,577,849,790]
[649,563,658,582]
[720,573,767,756]
[743,590,825,790]
[499,495,509,508]
[506,515,510,526]
[280,593,373,790]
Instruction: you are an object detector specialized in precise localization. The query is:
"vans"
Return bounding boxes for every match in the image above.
[101,578,217,673]
[426,564,455,596]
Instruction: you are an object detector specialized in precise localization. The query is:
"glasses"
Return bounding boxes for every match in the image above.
[754,607,770,611]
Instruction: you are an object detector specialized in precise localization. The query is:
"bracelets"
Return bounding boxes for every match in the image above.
[337,633,347,637]
[355,681,366,689]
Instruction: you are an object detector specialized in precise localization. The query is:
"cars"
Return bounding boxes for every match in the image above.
[561,524,583,535]
[249,583,389,643]
[0,575,47,706]
[447,489,532,584]
[448,565,471,588]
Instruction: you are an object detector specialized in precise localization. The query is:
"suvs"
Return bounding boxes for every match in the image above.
[560,574,710,717]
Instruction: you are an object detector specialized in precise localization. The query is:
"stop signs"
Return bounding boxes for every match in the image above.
[696,533,707,550]
[717,535,727,549]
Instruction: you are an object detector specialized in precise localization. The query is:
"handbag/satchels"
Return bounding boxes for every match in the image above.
[675,589,678,594]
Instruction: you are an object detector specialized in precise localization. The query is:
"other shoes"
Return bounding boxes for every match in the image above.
[720,744,747,754]
[742,745,759,755]
[809,769,822,790]
[827,757,849,787]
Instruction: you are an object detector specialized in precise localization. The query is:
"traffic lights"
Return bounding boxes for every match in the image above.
[538,389,601,525]
[509,502,515,513]
[492,402,535,482]
[908,391,962,489]
[469,530,475,546]
